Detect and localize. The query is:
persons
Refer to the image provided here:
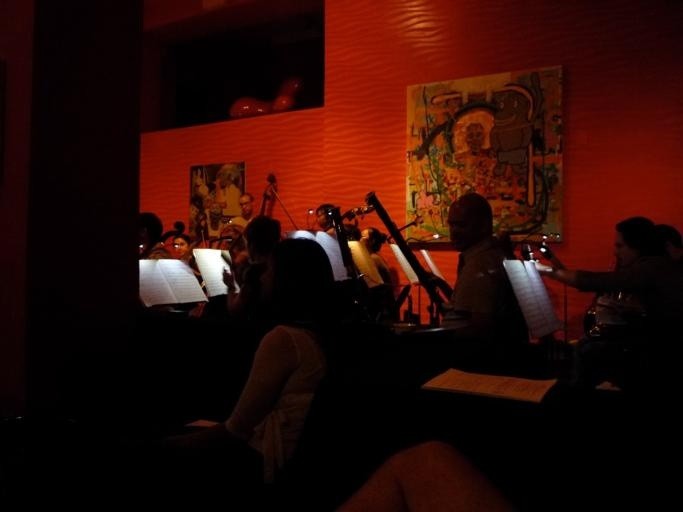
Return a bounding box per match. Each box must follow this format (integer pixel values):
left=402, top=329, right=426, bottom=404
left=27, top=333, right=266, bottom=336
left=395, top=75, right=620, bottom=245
left=137, top=193, right=683, bottom=512
